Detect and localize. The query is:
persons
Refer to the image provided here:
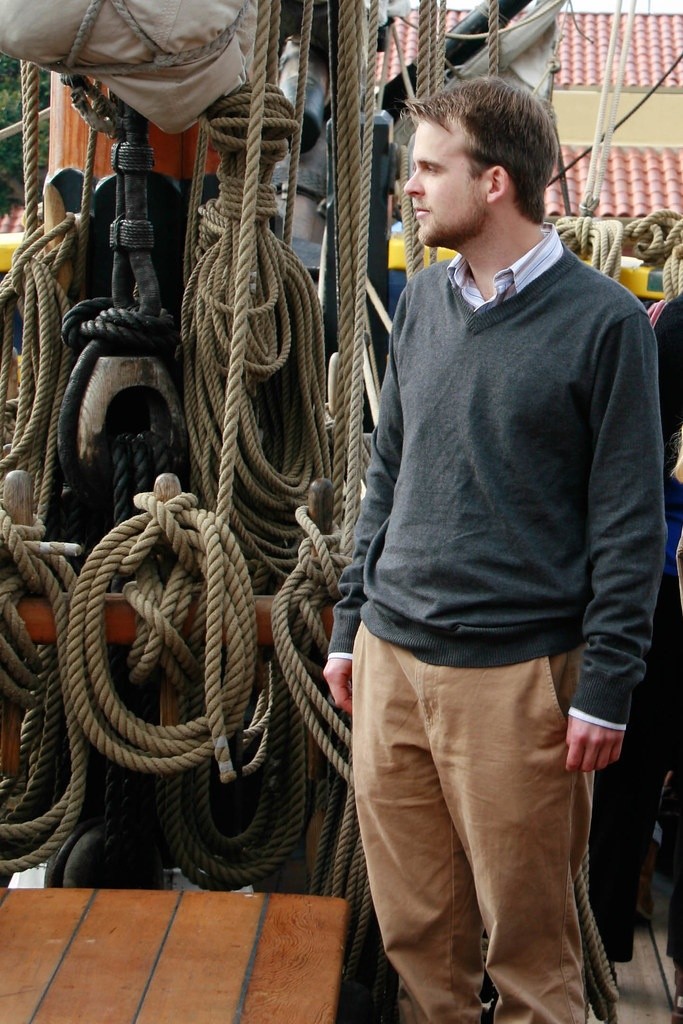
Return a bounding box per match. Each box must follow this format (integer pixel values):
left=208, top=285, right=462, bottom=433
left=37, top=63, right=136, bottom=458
left=591, top=296, right=683, bottom=1024
left=322, top=79, right=668, bottom=1024
